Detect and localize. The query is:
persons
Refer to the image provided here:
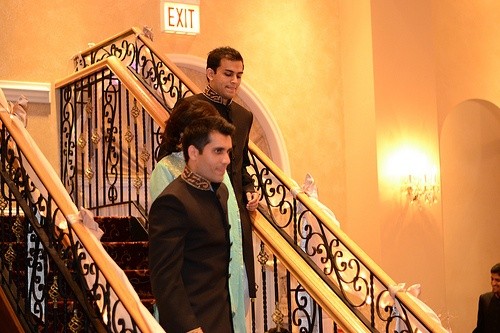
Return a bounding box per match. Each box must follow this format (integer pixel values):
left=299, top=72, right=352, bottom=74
left=147, top=99, right=250, bottom=333
left=145, top=116, right=234, bottom=333
left=471, top=263, right=500, bottom=333
left=158, top=46, right=259, bottom=230
